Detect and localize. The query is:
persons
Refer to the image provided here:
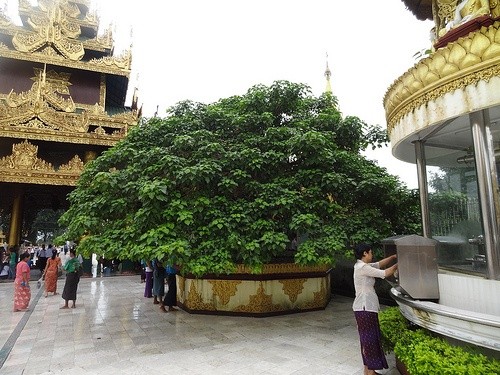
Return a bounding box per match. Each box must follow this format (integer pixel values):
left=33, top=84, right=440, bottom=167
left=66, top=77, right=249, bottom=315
left=352, top=242, right=399, bottom=375
left=0, top=241, right=179, bottom=313
